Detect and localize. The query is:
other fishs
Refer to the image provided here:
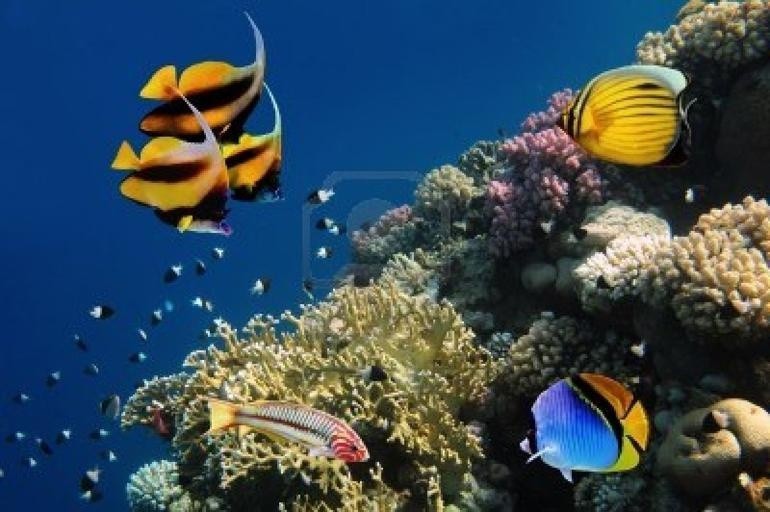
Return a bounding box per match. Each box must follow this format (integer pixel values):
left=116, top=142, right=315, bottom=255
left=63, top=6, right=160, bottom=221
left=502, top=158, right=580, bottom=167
left=163, top=298, right=175, bottom=314
left=79, top=489, right=104, bottom=504
left=684, top=188, right=693, bottom=203
left=86, top=304, right=117, bottom=321
left=152, top=309, right=163, bottom=328
left=328, top=224, right=347, bottom=236
left=96, top=393, right=120, bottom=418
left=491, top=328, right=502, bottom=343
left=23, top=457, right=37, bottom=468
left=78, top=469, right=101, bottom=490
left=92, top=427, right=111, bottom=440
left=137, top=328, right=149, bottom=343
left=518, top=372, right=653, bottom=483
left=191, top=295, right=205, bottom=310
left=163, top=261, right=184, bottom=283
left=138, top=9, right=267, bottom=144
left=206, top=397, right=371, bottom=463
left=247, top=277, right=270, bottom=297
left=315, top=246, right=333, bottom=260
left=4, top=430, right=26, bottom=443
left=540, top=218, right=555, bottom=235
left=210, top=247, right=225, bottom=260
left=35, top=437, right=53, bottom=455
left=0, top=467, right=5, bottom=479
left=301, top=279, right=316, bottom=300
left=556, top=63, right=698, bottom=169
left=354, top=367, right=388, bottom=382
left=630, top=338, right=646, bottom=357
left=56, top=428, right=73, bottom=445
left=211, top=82, right=283, bottom=205
left=194, top=258, right=207, bottom=277
left=204, top=300, right=213, bottom=312
left=110, top=79, right=231, bottom=237
left=73, top=333, right=89, bottom=351
left=130, top=352, right=149, bottom=364
left=45, top=371, right=61, bottom=389
left=452, top=220, right=467, bottom=232
left=99, top=449, right=119, bottom=464
left=306, top=186, right=336, bottom=205
left=501, top=327, right=513, bottom=342
left=12, top=391, right=31, bottom=406
left=84, top=362, right=99, bottom=374
left=314, top=217, right=334, bottom=231
